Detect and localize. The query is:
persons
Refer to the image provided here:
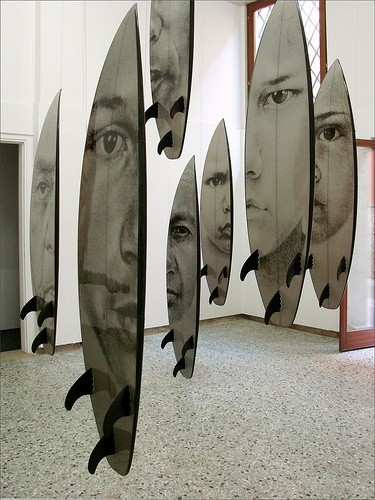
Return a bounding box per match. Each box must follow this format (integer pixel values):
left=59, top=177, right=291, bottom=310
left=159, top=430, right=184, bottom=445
left=30, top=90, right=59, bottom=354
left=204, top=122, right=232, bottom=303
left=308, top=56, right=354, bottom=310
left=79, top=6, right=140, bottom=471
left=151, top=1, right=192, bottom=159
left=165, top=158, right=201, bottom=377
left=244, top=1, right=311, bottom=328
